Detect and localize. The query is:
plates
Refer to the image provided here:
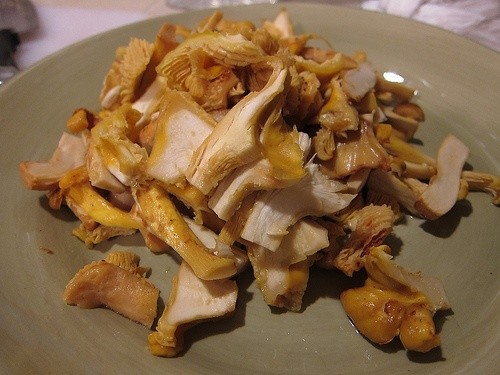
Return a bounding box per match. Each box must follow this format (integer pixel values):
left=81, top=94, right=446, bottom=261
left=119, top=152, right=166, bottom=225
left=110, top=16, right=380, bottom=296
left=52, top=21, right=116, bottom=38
left=0, top=2, right=498, bottom=374
